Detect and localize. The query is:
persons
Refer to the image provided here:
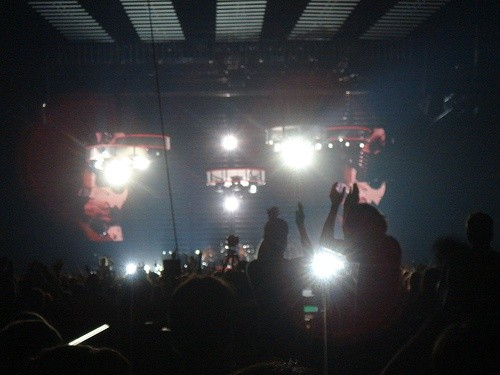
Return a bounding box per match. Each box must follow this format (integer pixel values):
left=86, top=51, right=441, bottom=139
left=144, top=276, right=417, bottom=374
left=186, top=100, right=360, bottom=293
left=0, top=184, right=499, bottom=373
